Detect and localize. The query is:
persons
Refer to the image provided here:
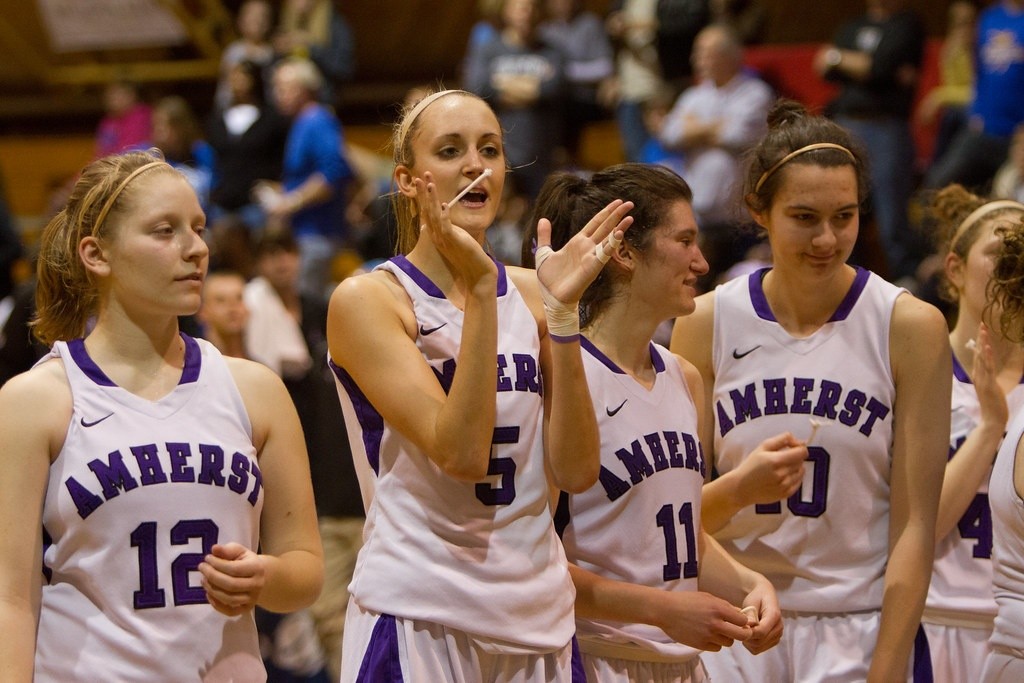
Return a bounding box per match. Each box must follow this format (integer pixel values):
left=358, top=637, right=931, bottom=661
left=910, top=182, right=1024, bottom=683
left=326, top=86, right=635, bottom=683
left=672, top=98, right=953, bottom=683
left=521, top=160, right=784, bottom=683
left=0, top=0, right=1024, bottom=514
left=0, top=148, right=327, bottom=683
